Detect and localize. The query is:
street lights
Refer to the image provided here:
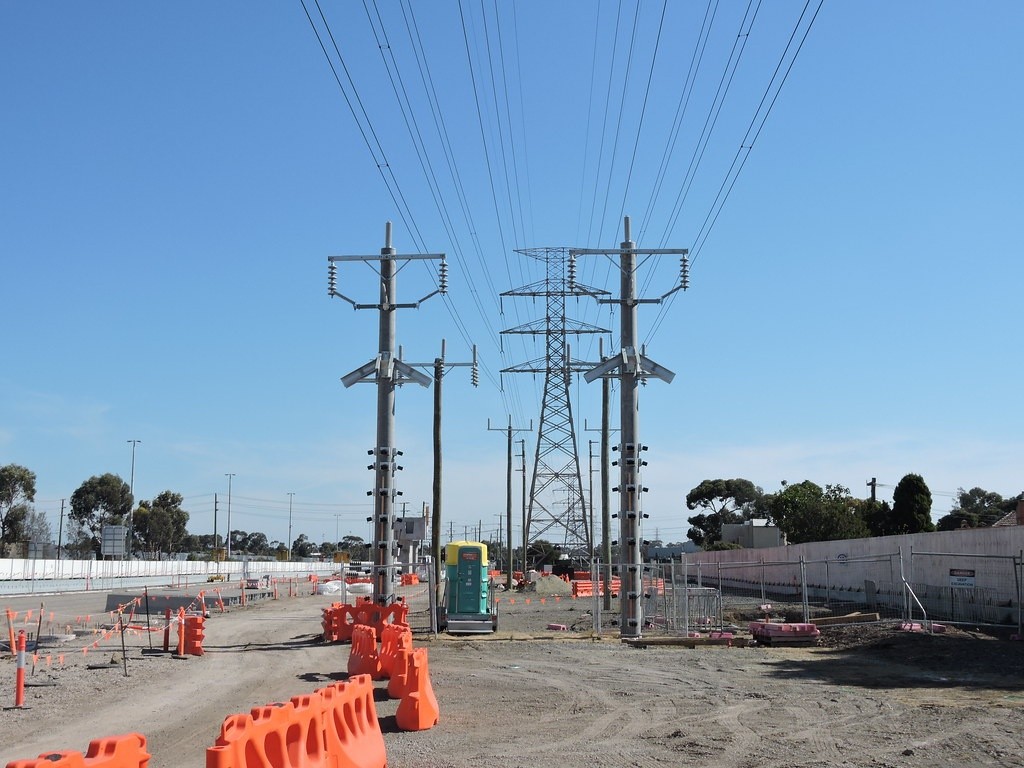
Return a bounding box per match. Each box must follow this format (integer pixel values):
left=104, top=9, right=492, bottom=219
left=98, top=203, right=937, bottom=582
left=127, top=440, right=142, bottom=561
left=224, top=473, right=236, bottom=559
left=287, top=492, right=296, bottom=561
left=334, top=514, right=341, bottom=551
